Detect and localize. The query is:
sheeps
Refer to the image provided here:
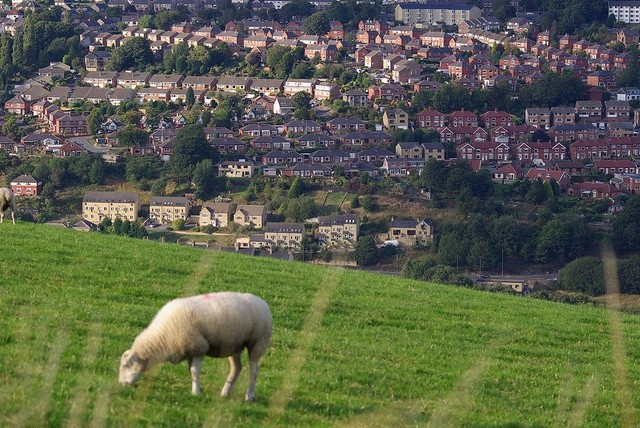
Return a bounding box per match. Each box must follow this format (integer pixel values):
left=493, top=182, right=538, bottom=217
left=0, top=187, right=16, bottom=225
left=118, top=291, right=274, bottom=403
